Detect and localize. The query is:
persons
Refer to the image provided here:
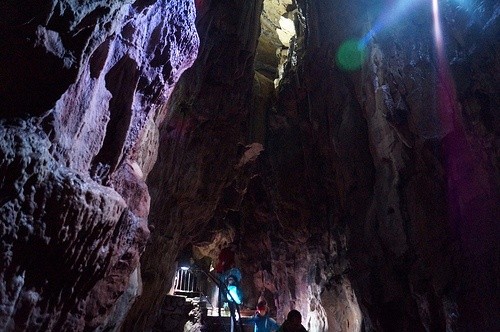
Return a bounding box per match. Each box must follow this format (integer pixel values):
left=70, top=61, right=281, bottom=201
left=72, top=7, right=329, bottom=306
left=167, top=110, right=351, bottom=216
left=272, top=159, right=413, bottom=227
left=275, top=309, right=307, bottom=332
left=224, top=276, right=243, bottom=309
left=238, top=302, right=279, bottom=332
